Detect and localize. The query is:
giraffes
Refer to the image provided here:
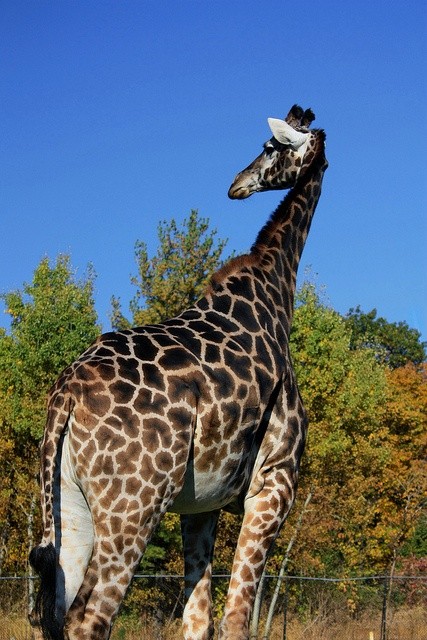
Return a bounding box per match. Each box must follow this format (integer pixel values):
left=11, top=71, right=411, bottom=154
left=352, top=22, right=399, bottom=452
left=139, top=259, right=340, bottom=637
left=29, top=105, right=328, bottom=639
